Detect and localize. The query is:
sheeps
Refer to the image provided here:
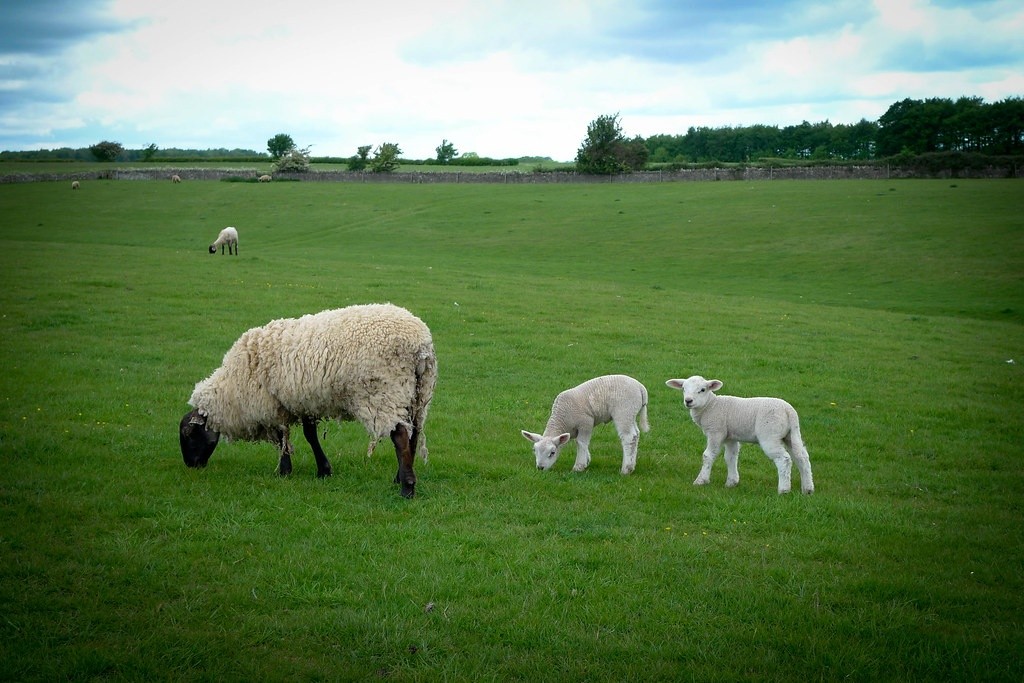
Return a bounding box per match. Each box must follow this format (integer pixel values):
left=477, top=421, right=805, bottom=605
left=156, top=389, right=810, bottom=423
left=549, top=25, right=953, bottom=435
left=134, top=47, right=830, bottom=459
left=172, top=176, right=181, bottom=184
left=258, top=174, right=272, bottom=182
left=521, top=374, right=652, bottom=475
left=666, top=376, right=815, bottom=495
left=72, top=180, right=80, bottom=189
left=209, top=227, right=238, bottom=256
left=178, top=304, right=434, bottom=498
